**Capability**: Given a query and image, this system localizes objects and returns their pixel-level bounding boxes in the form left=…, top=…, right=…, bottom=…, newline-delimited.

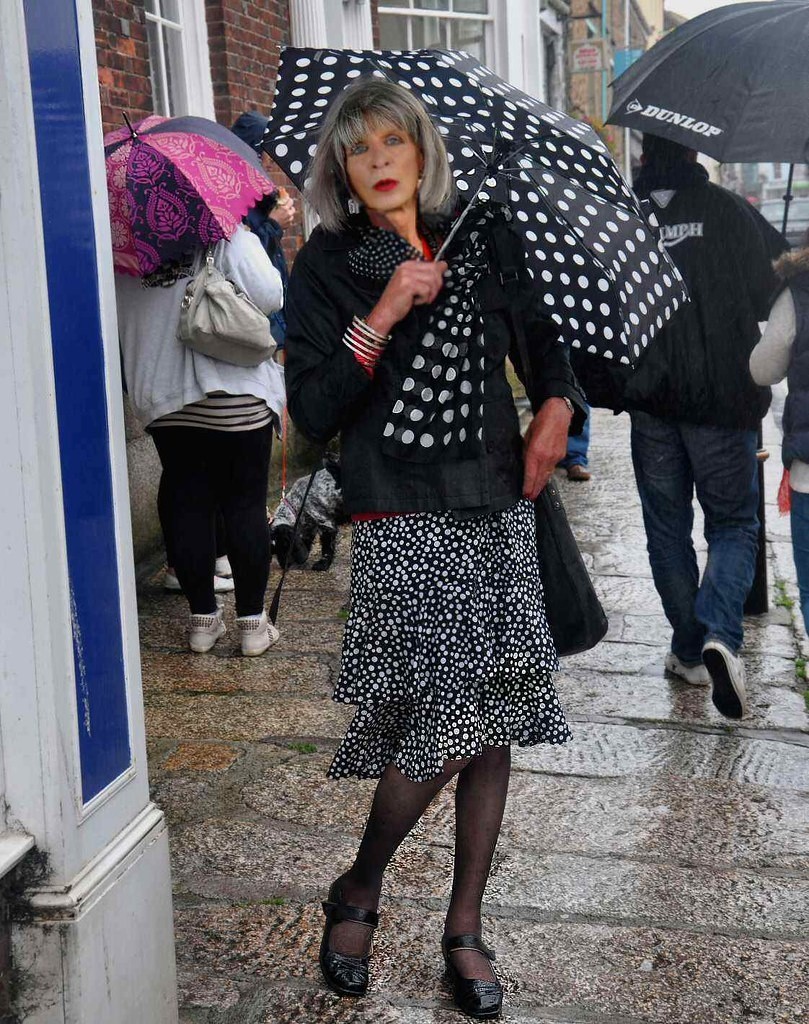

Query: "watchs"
left=564, top=397, right=574, bottom=416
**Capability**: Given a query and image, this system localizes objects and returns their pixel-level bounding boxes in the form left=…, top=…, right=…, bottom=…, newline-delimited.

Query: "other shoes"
left=163, top=554, right=235, bottom=592
left=567, top=464, right=591, bottom=481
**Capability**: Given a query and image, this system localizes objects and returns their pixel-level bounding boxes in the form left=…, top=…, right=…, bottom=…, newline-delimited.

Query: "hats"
left=230, top=111, right=270, bottom=157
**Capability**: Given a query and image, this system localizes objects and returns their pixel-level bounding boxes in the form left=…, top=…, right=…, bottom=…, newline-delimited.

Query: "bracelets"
left=343, top=315, right=391, bottom=367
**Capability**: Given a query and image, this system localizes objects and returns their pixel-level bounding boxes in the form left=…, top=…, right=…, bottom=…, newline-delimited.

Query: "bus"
left=761, top=180, right=809, bottom=201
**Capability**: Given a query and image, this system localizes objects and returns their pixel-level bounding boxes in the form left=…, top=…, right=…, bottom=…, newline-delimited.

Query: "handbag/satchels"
left=176, top=241, right=276, bottom=368
left=532, top=474, right=608, bottom=657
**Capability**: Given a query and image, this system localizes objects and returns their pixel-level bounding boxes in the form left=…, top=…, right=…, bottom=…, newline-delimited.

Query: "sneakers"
left=664, top=651, right=711, bottom=686
left=702, top=642, right=746, bottom=720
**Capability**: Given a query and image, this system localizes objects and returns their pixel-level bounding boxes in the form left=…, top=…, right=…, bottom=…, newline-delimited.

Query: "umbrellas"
left=256, top=45, right=693, bottom=372
left=601, top=0, right=808, bottom=236
left=103, top=115, right=274, bottom=285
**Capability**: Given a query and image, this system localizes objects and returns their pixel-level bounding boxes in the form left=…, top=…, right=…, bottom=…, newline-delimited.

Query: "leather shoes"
left=318, top=874, right=379, bottom=998
left=441, top=933, right=504, bottom=1019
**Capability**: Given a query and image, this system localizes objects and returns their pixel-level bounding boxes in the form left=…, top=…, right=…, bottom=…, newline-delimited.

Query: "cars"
left=758, top=199, right=809, bottom=248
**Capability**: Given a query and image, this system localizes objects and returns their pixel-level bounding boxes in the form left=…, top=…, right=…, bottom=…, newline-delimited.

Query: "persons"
left=117, top=111, right=296, bottom=656
left=750, top=228, right=809, bottom=635
left=556, top=406, right=590, bottom=480
left=628, top=132, right=792, bottom=719
left=284, top=84, right=590, bottom=1019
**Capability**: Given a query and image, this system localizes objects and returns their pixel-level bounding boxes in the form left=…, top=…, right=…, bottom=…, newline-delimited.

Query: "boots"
left=234, top=609, right=280, bottom=657
left=189, top=609, right=226, bottom=652
